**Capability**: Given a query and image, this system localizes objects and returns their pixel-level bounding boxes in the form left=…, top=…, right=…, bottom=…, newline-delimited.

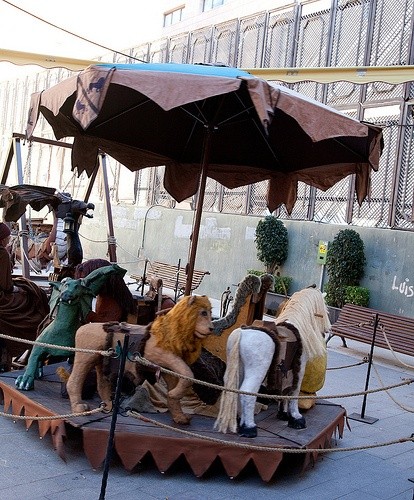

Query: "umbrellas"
left=26, top=63, right=385, bottom=296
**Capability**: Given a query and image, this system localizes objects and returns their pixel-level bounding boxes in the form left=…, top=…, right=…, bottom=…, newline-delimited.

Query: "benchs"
left=326, top=303, right=414, bottom=357
left=130, top=259, right=210, bottom=299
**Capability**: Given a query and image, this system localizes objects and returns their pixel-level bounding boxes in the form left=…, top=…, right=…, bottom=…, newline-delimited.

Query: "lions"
left=57, top=295, right=215, bottom=425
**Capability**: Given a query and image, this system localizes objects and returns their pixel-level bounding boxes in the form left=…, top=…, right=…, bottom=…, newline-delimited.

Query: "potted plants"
left=233, top=215, right=370, bottom=325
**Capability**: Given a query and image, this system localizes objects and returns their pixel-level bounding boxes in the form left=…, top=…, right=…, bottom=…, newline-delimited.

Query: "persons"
left=0, top=222, right=50, bottom=371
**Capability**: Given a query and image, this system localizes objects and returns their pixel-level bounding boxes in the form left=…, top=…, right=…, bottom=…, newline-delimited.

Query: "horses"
left=73, top=252, right=332, bottom=439
